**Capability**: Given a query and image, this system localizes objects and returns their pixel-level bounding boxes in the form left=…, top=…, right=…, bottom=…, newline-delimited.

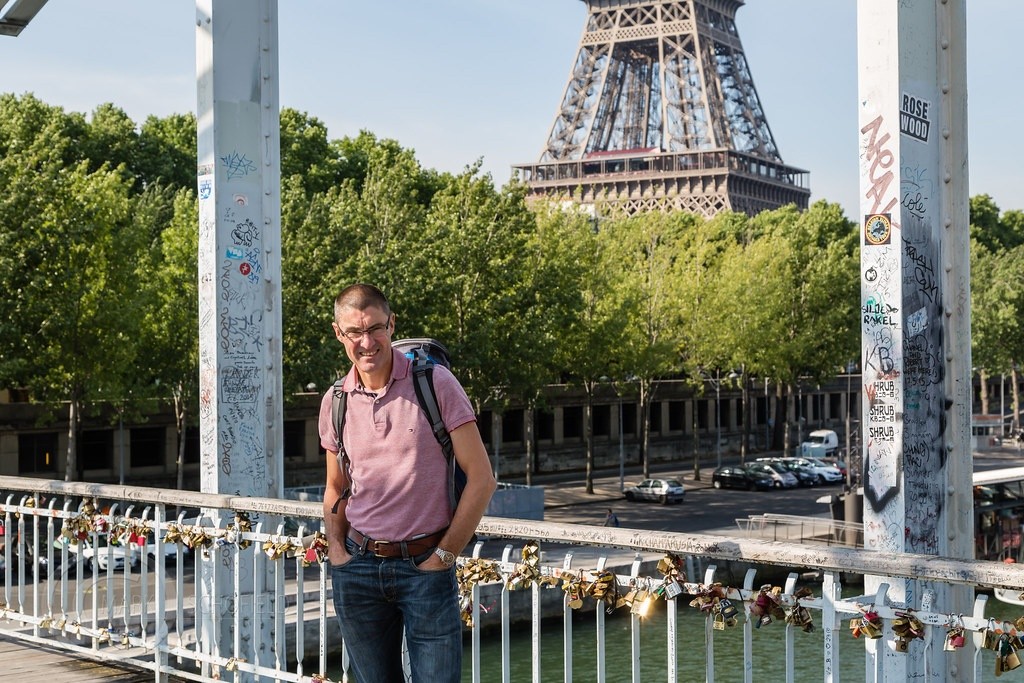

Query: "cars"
left=712, top=456, right=857, bottom=491
left=0, top=520, right=191, bottom=582
left=622, top=478, right=686, bottom=505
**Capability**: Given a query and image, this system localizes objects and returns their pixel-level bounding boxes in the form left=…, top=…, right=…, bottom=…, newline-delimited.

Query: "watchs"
left=434, top=547, right=456, bottom=568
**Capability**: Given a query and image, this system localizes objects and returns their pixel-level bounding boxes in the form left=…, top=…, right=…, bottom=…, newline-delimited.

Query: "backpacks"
left=331, top=337, right=468, bottom=516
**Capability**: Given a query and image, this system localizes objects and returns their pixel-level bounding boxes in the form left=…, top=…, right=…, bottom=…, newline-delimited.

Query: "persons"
left=605, top=508, right=618, bottom=528
left=317, top=284, right=499, bottom=683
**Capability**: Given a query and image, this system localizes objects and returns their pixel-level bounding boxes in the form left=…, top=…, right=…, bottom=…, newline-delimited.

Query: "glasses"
left=335, top=313, right=392, bottom=342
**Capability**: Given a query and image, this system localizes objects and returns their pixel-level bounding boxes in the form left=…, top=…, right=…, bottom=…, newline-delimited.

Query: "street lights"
left=698, top=370, right=738, bottom=469
left=599, top=376, right=645, bottom=493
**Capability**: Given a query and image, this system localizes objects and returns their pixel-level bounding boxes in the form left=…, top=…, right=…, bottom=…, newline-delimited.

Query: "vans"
left=806, top=429, right=839, bottom=455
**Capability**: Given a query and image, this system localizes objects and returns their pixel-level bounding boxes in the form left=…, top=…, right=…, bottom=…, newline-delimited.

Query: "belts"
left=347, top=525, right=449, bottom=558
